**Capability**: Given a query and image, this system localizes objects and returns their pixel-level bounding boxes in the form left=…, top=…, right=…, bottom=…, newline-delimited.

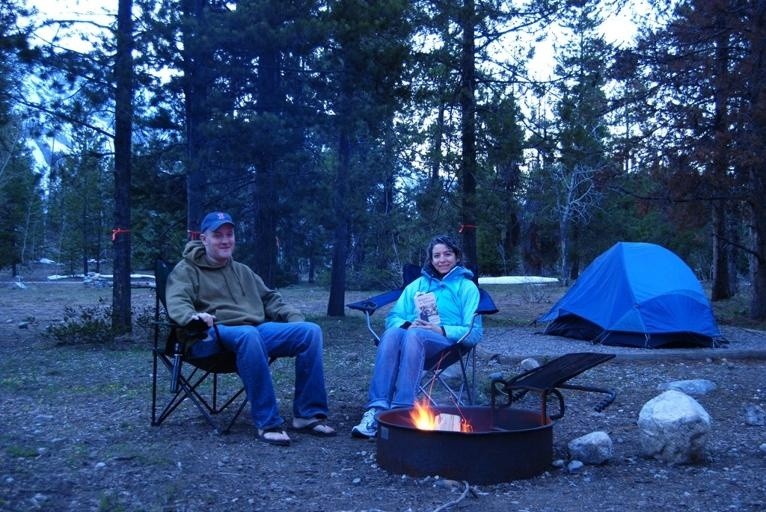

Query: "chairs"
left=345, top=262, right=498, bottom=407
left=149, top=252, right=278, bottom=437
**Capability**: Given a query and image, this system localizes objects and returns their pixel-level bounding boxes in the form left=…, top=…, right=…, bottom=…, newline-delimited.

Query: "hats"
left=200, top=211, right=235, bottom=233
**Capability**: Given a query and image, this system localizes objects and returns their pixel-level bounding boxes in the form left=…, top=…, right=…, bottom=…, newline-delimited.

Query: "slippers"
left=257, top=426, right=292, bottom=446
left=287, top=417, right=338, bottom=438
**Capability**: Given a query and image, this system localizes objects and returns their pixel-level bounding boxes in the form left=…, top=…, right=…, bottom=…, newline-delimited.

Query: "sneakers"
left=350, top=407, right=379, bottom=440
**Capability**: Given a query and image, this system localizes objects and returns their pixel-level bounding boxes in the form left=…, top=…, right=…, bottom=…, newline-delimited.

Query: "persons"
left=351, top=235, right=484, bottom=439
left=165, top=212, right=338, bottom=448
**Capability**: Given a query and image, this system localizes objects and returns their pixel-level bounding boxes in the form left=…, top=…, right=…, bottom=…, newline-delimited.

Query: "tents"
left=528, top=242, right=730, bottom=351
